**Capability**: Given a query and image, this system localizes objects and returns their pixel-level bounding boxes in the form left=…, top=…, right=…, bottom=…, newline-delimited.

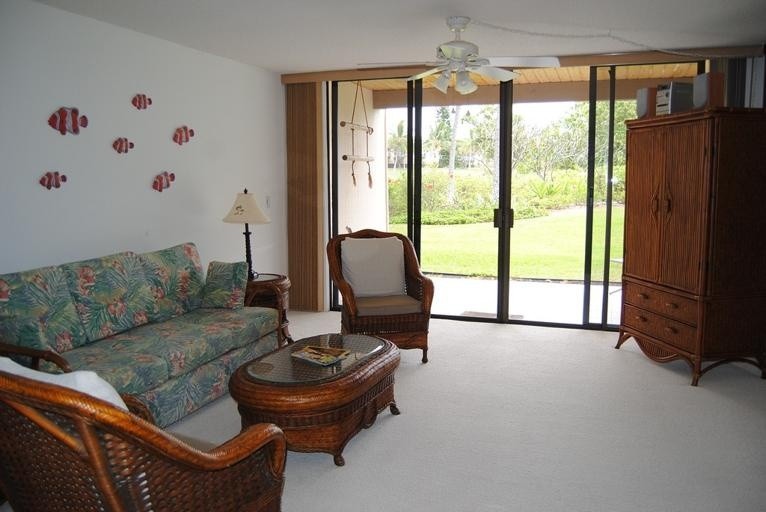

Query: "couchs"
left=0, top=282, right=290, bottom=429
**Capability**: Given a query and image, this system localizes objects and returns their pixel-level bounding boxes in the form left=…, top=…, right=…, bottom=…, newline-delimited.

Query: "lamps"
left=407, top=17, right=520, bottom=96
left=223, top=188, right=271, bottom=281
left=440, top=16, right=480, bottom=63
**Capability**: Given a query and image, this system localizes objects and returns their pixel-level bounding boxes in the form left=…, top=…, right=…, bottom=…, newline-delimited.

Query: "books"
left=292, top=346, right=351, bottom=367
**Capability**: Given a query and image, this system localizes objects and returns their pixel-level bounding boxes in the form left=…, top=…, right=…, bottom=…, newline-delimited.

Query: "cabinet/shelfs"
left=614, top=107, right=766, bottom=386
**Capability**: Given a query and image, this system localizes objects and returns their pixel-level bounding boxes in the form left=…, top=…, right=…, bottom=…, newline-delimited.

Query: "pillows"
left=137, top=242, right=206, bottom=324
left=340, top=236, right=406, bottom=299
left=1, top=356, right=154, bottom=508
left=60, top=251, right=162, bottom=345
left=0, top=264, right=87, bottom=368
left=201, top=261, right=249, bottom=310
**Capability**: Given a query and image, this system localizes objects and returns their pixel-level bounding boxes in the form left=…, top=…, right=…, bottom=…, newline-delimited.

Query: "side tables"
left=247, top=273, right=295, bottom=344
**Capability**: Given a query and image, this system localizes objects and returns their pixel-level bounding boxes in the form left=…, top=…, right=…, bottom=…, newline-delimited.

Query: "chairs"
left=326, top=229, right=434, bottom=363
left=0, top=371, right=287, bottom=512
